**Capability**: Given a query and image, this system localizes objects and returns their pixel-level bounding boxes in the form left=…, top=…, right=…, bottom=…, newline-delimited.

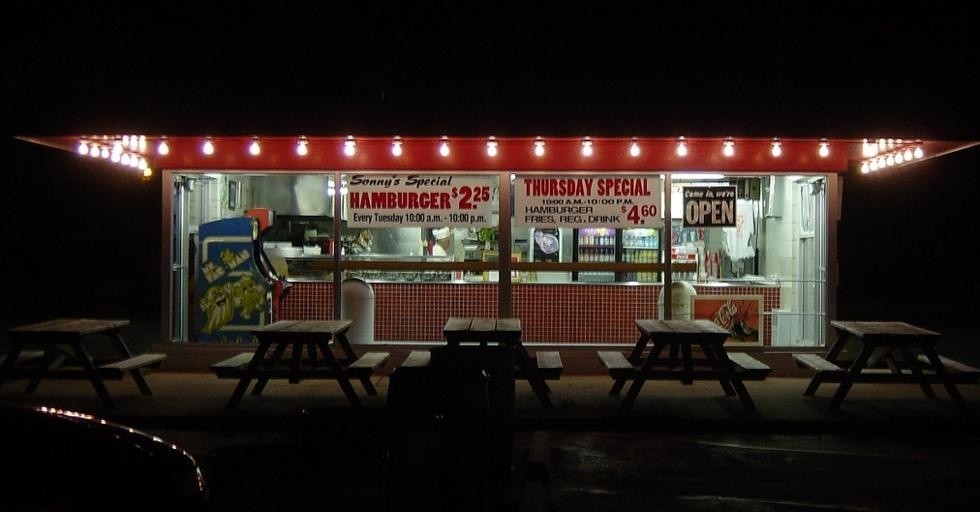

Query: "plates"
left=263, top=242, right=321, bottom=257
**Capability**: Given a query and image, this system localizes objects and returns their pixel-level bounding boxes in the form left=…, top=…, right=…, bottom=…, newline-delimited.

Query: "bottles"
left=579, top=248, right=659, bottom=264
left=579, top=228, right=657, bottom=249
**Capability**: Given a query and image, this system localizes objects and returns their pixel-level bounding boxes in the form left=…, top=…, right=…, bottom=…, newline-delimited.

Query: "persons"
left=428, top=228, right=451, bottom=256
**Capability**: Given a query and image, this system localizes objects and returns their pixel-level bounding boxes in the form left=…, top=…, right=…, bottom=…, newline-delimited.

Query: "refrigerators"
left=571, top=225, right=662, bottom=284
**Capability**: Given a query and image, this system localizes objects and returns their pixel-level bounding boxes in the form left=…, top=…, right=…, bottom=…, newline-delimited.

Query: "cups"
left=705, top=249, right=718, bottom=278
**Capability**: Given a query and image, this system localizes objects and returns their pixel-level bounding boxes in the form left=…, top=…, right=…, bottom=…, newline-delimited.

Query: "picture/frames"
left=690, top=294, right=764, bottom=347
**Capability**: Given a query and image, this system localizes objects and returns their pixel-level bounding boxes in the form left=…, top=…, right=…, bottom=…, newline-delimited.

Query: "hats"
left=433, top=227, right=450, bottom=240
